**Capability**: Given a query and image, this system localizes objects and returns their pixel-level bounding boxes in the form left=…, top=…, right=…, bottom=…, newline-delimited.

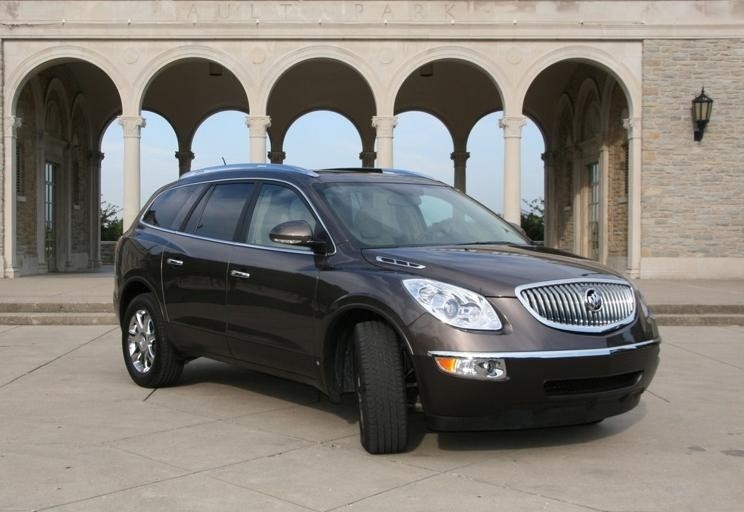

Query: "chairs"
left=353, top=208, right=407, bottom=247
left=253, top=205, right=320, bottom=250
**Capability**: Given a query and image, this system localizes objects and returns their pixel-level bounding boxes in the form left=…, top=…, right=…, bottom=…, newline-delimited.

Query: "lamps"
left=419, top=63, right=434, bottom=76
left=692, top=85, right=714, bottom=141
left=209, top=62, right=222, bottom=76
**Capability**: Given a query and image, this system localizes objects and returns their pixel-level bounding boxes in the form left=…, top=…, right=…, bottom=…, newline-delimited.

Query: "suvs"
left=113, top=158, right=660, bottom=456
